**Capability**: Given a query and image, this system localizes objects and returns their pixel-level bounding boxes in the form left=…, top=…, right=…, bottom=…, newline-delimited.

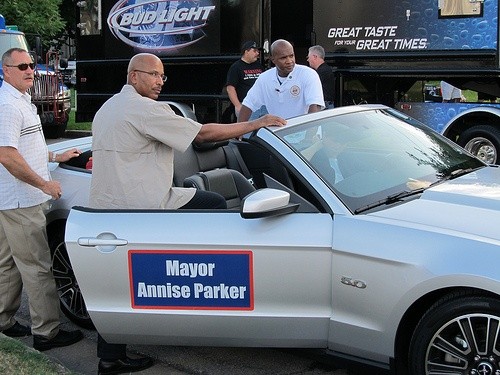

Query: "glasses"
left=134, top=69, right=167, bottom=82
left=6, top=63, right=36, bottom=71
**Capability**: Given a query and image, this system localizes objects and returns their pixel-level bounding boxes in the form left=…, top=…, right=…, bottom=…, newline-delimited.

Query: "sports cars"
left=45, top=103, right=500, bottom=375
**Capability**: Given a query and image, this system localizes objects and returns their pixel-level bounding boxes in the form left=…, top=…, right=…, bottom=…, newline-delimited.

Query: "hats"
left=240, top=41, right=266, bottom=52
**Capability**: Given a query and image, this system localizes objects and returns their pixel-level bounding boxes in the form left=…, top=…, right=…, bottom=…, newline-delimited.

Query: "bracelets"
left=52, top=152, right=57, bottom=163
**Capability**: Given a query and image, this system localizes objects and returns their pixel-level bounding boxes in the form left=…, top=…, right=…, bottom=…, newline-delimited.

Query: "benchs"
left=169, top=142, right=259, bottom=198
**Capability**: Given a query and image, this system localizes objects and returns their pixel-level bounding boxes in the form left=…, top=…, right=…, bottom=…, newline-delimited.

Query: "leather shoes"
left=33, top=329, right=83, bottom=352
left=2, top=321, right=32, bottom=337
left=97, top=354, right=154, bottom=375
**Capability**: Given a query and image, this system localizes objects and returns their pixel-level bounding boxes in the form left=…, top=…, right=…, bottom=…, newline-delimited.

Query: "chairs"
left=183, top=168, right=258, bottom=210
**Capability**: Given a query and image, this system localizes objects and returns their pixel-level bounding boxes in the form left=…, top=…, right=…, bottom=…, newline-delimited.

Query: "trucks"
left=71, top=0, right=500, bottom=165
left=0, top=13, right=72, bottom=139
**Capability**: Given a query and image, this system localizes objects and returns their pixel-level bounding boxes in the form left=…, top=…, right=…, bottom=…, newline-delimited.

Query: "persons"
left=441, top=81, right=466, bottom=103
left=307, top=45, right=335, bottom=110
left=0, top=48, right=83, bottom=351
left=231, top=39, right=326, bottom=143
left=89, top=53, right=287, bottom=375
left=225, top=40, right=265, bottom=123
